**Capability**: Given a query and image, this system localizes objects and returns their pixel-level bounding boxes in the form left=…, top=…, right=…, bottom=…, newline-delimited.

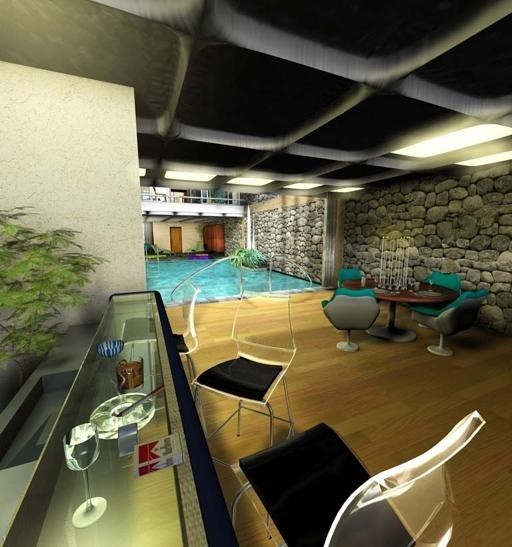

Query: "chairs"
left=122, top=278, right=202, bottom=408
left=150, top=244, right=177, bottom=255
left=185, top=239, right=201, bottom=255
left=320, top=285, right=380, bottom=354
left=152, top=186, right=166, bottom=201
left=398, top=270, right=462, bottom=328
left=410, top=286, right=489, bottom=358
left=335, top=266, right=367, bottom=289
left=188, top=286, right=301, bottom=470
left=230, top=407, right=488, bottom=547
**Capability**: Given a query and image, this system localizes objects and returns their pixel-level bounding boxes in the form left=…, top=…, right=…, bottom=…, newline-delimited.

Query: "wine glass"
left=62, top=422, right=109, bottom=528
left=408, top=278, right=415, bottom=290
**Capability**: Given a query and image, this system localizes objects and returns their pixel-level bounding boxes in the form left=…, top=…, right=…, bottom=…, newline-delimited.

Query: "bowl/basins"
left=96, top=339, right=124, bottom=357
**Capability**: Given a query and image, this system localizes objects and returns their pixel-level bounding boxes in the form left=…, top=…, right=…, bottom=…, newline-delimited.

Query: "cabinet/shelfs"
left=203, top=222, right=227, bottom=257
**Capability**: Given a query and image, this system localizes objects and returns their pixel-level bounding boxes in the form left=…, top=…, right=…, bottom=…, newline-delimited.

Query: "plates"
left=89, top=392, right=156, bottom=439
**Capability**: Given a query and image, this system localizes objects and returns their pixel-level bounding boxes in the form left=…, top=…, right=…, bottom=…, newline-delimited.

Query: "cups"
left=115, top=354, right=145, bottom=394
left=414, top=281, right=420, bottom=291
left=360, top=276, right=366, bottom=287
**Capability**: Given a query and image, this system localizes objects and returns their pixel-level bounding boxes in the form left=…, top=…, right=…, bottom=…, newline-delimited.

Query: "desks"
left=341, top=277, right=460, bottom=346
